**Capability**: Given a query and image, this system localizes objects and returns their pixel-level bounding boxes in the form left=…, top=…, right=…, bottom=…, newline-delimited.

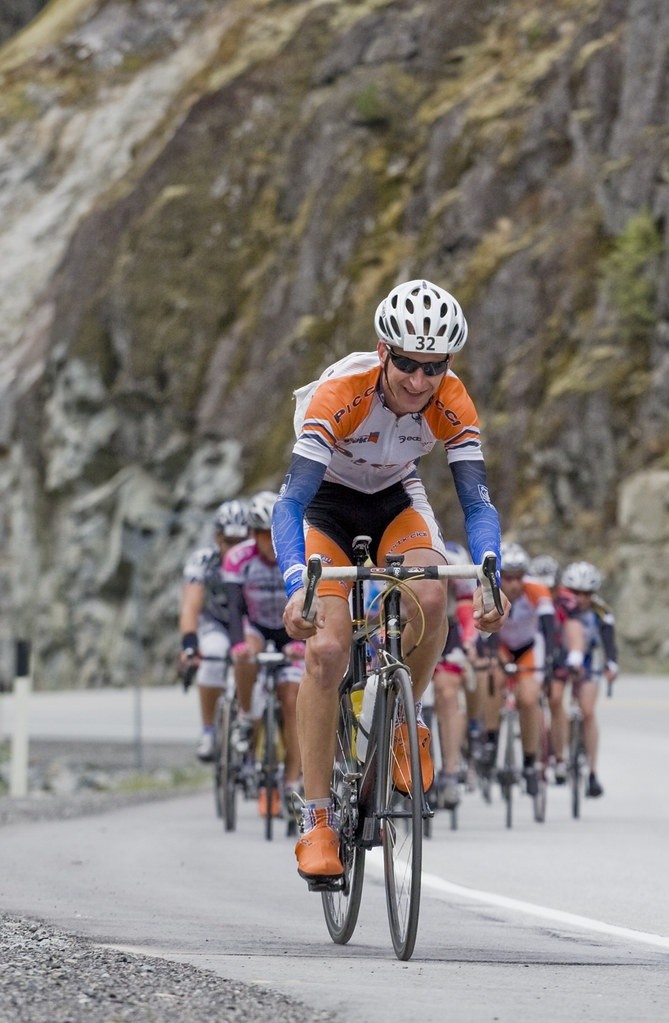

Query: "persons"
left=179, top=493, right=618, bottom=834
left=269, top=276, right=512, bottom=885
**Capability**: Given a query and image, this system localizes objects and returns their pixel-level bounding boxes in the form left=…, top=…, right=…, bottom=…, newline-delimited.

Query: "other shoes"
left=258, top=784, right=280, bottom=816
left=589, top=777, right=602, bottom=795
left=294, top=811, right=345, bottom=876
left=230, top=723, right=251, bottom=751
left=396, top=715, right=435, bottom=794
left=200, top=733, right=218, bottom=757
left=437, top=778, right=459, bottom=806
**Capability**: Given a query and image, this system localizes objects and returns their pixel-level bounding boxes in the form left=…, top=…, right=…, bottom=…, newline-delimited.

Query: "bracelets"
left=182, top=633, right=197, bottom=650
left=564, top=650, right=583, bottom=669
left=607, top=660, right=618, bottom=673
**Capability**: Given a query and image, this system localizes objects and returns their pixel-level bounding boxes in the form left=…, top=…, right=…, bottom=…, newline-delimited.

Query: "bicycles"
left=181, top=652, right=616, bottom=840
left=301, top=533, right=503, bottom=960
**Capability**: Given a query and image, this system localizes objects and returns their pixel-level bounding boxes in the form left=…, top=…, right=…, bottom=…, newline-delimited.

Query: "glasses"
left=387, top=343, right=450, bottom=377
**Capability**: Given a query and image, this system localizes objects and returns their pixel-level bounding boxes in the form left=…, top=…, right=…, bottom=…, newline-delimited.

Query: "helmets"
left=445, top=543, right=602, bottom=591
left=214, top=499, right=248, bottom=534
left=247, top=490, right=278, bottom=529
left=375, top=278, right=469, bottom=355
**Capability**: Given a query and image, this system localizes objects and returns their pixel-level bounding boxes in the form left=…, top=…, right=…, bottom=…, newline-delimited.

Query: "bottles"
left=350, top=674, right=379, bottom=762
